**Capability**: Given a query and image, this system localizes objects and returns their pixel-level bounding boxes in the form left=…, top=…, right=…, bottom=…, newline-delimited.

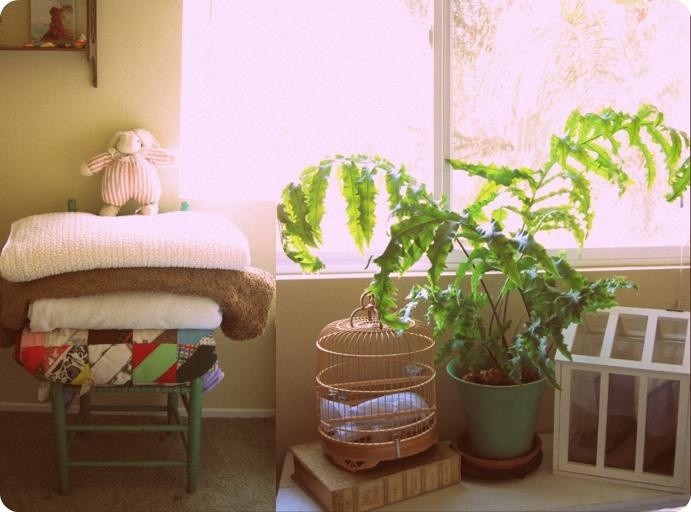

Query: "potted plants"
left=278, top=102, right=691, bottom=460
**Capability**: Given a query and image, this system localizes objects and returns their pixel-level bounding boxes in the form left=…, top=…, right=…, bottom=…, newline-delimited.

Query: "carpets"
left=0, top=411, right=277, bottom=512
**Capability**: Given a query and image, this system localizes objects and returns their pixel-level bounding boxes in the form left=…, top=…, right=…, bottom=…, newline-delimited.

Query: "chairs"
left=49, top=198, right=203, bottom=495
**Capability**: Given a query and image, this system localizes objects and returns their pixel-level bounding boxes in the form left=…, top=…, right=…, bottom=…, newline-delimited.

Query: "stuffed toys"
left=77, top=125, right=177, bottom=216
